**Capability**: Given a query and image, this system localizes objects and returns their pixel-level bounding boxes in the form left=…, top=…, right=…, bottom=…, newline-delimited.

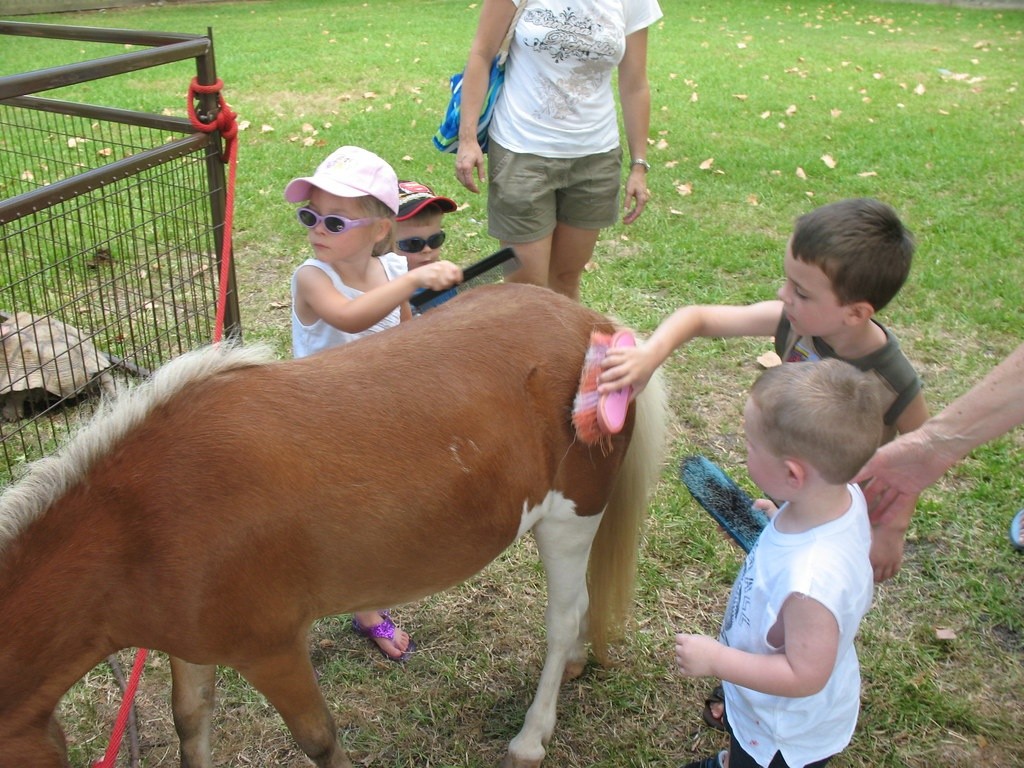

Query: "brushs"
left=409, top=246, right=523, bottom=315
left=679, top=454, right=771, bottom=554
left=571, top=329, right=638, bottom=453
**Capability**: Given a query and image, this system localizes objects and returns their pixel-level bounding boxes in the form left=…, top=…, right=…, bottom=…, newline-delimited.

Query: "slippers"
left=351, top=609, right=415, bottom=660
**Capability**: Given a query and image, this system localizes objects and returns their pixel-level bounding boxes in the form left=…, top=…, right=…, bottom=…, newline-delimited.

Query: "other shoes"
left=704, top=682, right=728, bottom=731
left=679, top=749, right=729, bottom=768
left=1010, top=508, right=1024, bottom=549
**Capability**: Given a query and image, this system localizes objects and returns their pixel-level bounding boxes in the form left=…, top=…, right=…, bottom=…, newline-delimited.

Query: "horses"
left=1, top=284, right=672, bottom=768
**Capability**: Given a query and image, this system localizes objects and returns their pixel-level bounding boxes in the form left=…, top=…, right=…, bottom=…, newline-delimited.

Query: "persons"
left=596, top=197, right=930, bottom=585
left=291, top=145, right=463, bottom=660
left=680, top=357, right=874, bottom=768
left=850, top=341, right=1024, bottom=528
left=455, top=0, right=664, bottom=304
left=1009, top=509, right=1024, bottom=552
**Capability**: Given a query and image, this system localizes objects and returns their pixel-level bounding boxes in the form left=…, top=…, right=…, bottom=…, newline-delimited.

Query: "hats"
left=394, top=180, right=457, bottom=221
left=285, top=146, right=399, bottom=215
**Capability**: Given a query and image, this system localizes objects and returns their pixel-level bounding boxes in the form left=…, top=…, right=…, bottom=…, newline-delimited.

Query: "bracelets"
left=630, top=159, right=651, bottom=173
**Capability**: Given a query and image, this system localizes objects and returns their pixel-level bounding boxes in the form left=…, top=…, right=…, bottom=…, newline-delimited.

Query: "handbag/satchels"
left=432, top=0, right=531, bottom=154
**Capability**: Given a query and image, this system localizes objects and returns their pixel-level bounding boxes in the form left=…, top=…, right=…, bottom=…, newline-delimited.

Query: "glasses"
left=296, top=204, right=380, bottom=234
left=394, top=230, right=446, bottom=253
